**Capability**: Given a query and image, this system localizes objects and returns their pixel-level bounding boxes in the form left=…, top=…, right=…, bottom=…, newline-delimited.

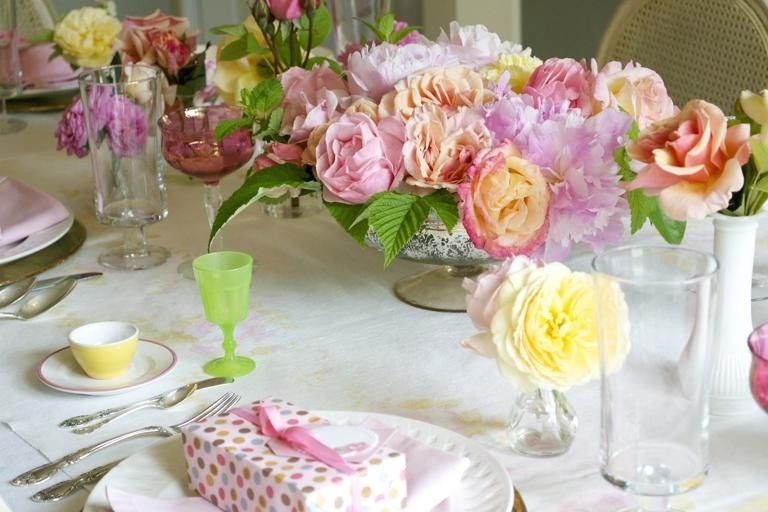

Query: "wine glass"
left=75, top=64, right=175, bottom=272
left=191, top=250, right=257, bottom=377
left=158, top=102, right=254, bottom=279
left=0, top=0, right=26, bottom=137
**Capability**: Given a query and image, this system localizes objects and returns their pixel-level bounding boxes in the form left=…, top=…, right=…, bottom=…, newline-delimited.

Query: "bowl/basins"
left=66, top=320, right=136, bottom=380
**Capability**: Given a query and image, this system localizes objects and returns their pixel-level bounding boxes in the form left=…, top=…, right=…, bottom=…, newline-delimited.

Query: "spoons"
left=0, top=277, right=81, bottom=324
left=75, top=381, right=201, bottom=435
left=0, top=274, right=40, bottom=307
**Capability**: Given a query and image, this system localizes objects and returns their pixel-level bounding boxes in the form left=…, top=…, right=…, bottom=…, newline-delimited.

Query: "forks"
left=12, top=390, right=240, bottom=489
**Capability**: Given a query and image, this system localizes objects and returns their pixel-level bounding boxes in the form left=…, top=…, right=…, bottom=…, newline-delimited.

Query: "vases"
left=501, top=377, right=582, bottom=459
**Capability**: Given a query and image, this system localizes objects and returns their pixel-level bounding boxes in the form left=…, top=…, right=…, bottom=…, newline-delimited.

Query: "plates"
left=7, top=79, right=96, bottom=105
left=1, top=200, right=72, bottom=266
left=37, top=339, right=179, bottom=396
left=81, top=404, right=515, bottom=512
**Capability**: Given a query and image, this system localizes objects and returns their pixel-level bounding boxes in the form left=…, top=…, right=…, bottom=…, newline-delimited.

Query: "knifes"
left=32, top=271, right=107, bottom=291
left=59, top=376, right=235, bottom=430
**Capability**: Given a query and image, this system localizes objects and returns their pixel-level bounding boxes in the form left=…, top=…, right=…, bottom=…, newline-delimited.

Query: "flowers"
left=462, top=252, right=633, bottom=393
left=52, top=0, right=766, bottom=266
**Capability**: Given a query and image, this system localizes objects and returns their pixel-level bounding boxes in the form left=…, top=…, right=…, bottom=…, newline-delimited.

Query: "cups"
left=746, top=319, right=768, bottom=413
left=592, top=242, right=722, bottom=499
